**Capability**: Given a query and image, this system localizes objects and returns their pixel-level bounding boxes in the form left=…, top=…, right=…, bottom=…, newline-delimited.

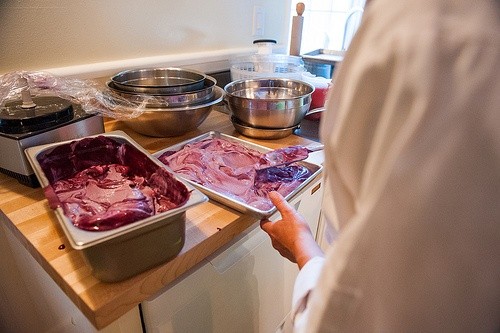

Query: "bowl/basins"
left=95, top=67, right=225, bottom=137
left=24, top=129, right=209, bottom=283
left=224, top=77, right=316, bottom=129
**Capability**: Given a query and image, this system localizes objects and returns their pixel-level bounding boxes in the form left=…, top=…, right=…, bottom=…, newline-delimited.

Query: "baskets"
left=230, top=61, right=306, bottom=81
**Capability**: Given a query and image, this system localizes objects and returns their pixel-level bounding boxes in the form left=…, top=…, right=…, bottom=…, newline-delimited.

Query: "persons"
left=260, top=0, right=500, bottom=333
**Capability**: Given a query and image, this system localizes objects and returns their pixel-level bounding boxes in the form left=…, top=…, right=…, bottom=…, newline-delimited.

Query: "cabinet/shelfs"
left=0, top=162, right=324, bottom=333
